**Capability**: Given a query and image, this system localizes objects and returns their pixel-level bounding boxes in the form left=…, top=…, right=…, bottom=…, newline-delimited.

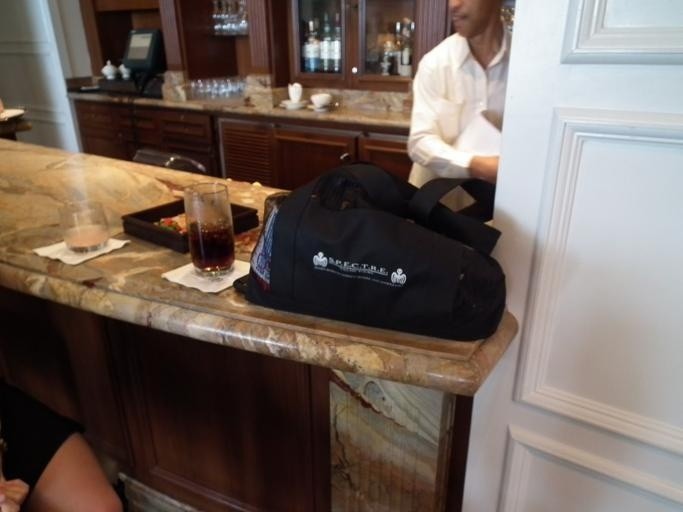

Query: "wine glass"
left=210, top=0, right=248, bottom=35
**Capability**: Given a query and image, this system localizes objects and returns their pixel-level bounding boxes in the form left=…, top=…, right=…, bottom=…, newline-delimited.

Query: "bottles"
left=319, top=12, right=331, bottom=72
left=303, top=20, right=319, bottom=70
left=330, top=14, right=341, bottom=72
left=100, top=60, right=117, bottom=80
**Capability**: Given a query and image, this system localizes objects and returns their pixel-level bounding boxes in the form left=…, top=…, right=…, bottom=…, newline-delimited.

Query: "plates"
left=281, top=99, right=307, bottom=109
left=308, top=105, right=332, bottom=112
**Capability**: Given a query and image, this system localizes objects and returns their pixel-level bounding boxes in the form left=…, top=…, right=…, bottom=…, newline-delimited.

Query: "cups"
left=311, top=94, right=329, bottom=107
left=184, top=183, right=240, bottom=277
left=188, top=76, right=244, bottom=98
left=118, top=63, right=131, bottom=79
left=59, top=202, right=109, bottom=252
left=288, top=83, right=302, bottom=102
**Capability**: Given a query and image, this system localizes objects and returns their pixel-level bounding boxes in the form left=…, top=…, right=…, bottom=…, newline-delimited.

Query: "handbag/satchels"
left=246, top=160, right=505, bottom=340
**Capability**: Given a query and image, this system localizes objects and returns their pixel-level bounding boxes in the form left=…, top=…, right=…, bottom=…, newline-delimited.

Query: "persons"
left=1, top=375, right=125, bottom=512
left=403, top=1, right=513, bottom=222
